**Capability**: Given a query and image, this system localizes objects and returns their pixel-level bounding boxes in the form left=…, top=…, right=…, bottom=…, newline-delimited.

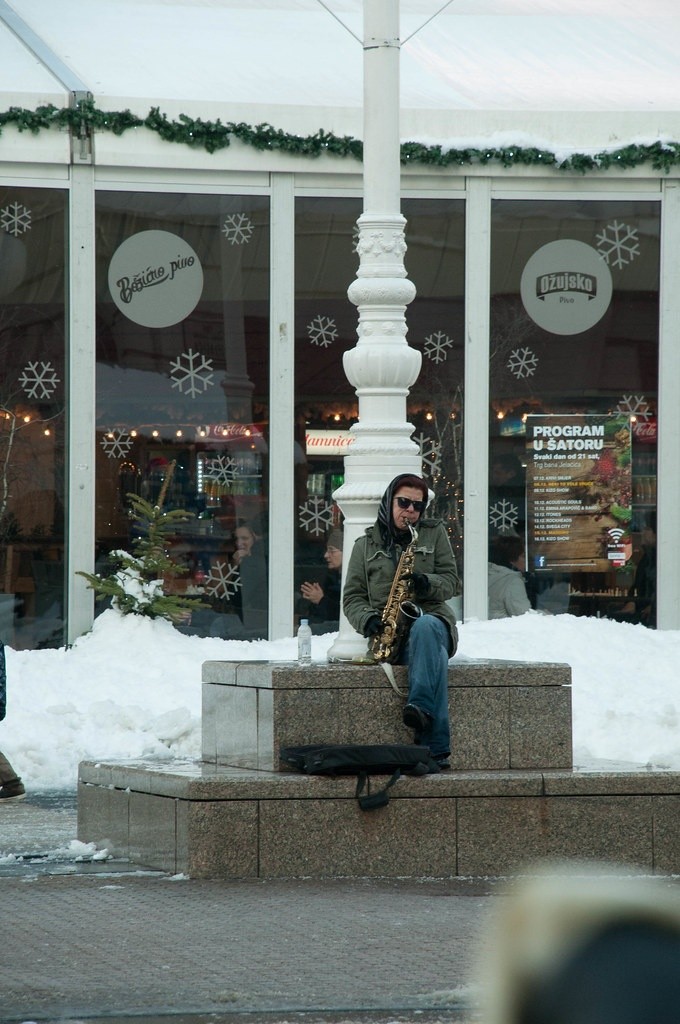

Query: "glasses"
left=393, top=496, right=425, bottom=512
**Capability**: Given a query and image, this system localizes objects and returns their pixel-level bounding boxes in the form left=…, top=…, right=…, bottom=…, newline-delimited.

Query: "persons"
left=614, top=510, right=657, bottom=630
left=487, top=534, right=536, bottom=621
left=230, top=522, right=268, bottom=570
left=0, top=639, right=25, bottom=800
left=343, top=472, right=458, bottom=769
left=463, top=859, right=680, bottom=1024
left=301, top=528, right=345, bottom=624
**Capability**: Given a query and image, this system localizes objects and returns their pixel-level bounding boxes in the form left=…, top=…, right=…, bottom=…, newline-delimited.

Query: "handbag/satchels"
left=279, top=743, right=432, bottom=809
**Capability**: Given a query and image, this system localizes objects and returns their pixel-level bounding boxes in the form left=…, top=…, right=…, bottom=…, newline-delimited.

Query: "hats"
left=326, top=529, right=343, bottom=550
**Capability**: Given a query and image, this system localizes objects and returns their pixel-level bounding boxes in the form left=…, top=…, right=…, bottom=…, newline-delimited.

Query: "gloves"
left=399, top=573, right=429, bottom=597
left=364, top=618, right=385, bottom=638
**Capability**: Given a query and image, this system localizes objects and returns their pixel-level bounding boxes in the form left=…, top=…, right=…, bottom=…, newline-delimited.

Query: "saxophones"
left=370, top=517, right=425, bottom=660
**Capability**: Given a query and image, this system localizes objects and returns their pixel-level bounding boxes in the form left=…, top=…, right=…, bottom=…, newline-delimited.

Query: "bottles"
left=297, top=619, right=312, bottom=666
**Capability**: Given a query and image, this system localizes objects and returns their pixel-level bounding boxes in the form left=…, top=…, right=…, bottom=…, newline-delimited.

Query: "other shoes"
left=0, top=777, right=26, bottom=798
left=403, top=703, right=430, bottom=734
left=433, top=755, right=451, bottom=769
